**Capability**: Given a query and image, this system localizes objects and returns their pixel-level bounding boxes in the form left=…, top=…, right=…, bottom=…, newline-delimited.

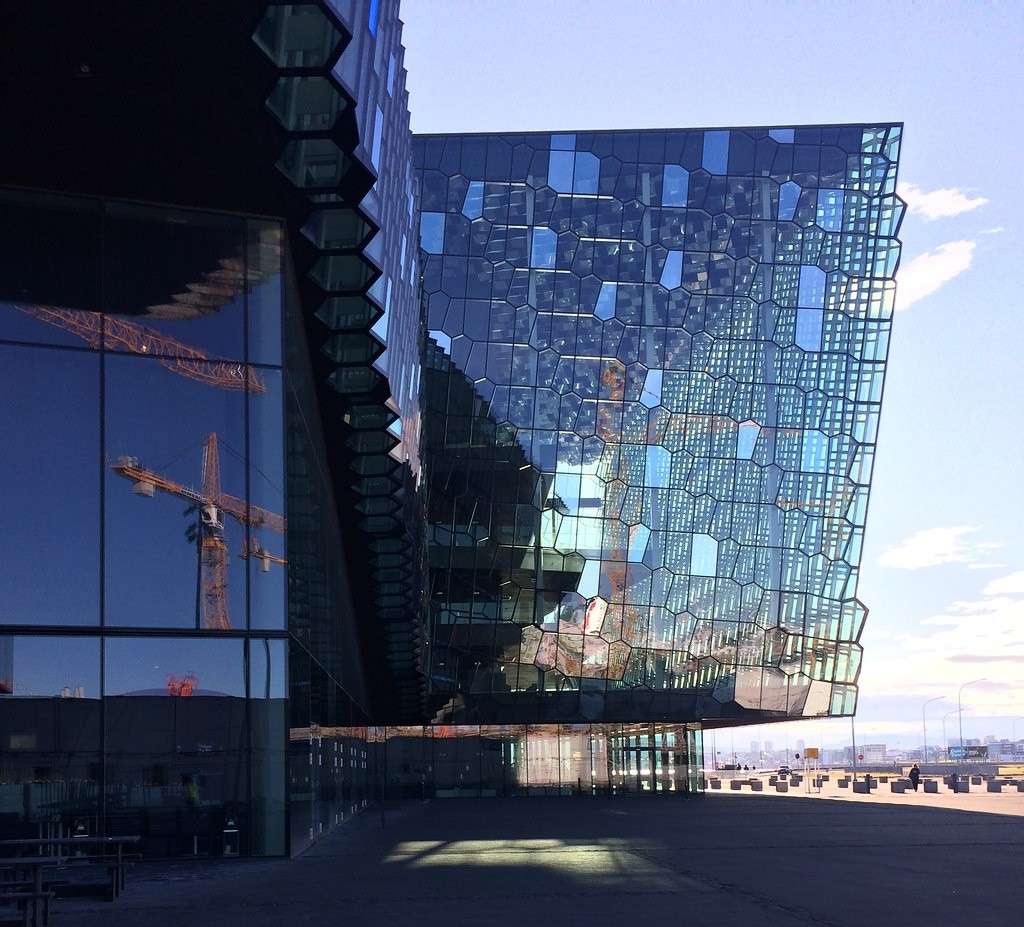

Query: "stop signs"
left=858, top=755, right=864, bottom=760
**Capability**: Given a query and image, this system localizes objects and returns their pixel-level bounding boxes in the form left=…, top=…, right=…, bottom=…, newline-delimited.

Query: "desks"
left=0, top=856, right=67, bottom=922
left=0, top=835, right=141, bottom=898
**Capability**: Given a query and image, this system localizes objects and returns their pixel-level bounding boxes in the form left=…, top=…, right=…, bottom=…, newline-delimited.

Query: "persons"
left=744, top=765, right=749, bottom=770
left=909, top=764, right=920, bottom=792
left=737, top=763, right=741, bottom=770
left=182, top=773, right=201, bottom=807
left=752, top=765, right=756, bottom=770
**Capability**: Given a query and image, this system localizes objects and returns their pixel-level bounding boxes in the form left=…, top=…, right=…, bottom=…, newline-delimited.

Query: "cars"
left=778, top=765, right=793, bottom=775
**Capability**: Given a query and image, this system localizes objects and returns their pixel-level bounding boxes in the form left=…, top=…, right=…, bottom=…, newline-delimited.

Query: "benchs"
left=1, top=861, right=135, bottom=903
left=0, top=876, right=72, bottom=916
left=66, top=853, right=143, bottom=891
left=0, top=891, right=55, bottom=926
left=435, top=787, right=497, bottom=797
left=518, top=787, right=572, bottom=796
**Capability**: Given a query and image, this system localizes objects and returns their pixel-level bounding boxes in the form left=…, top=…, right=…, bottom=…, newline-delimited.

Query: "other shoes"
left=913, top=787, right=917, bottom=792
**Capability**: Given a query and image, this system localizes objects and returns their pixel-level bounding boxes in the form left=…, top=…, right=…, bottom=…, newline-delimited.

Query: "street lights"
left=922, top=696, right=945, bottom=763
left=943, top=709, right=965, bottom=762
left=959, top=679, right=988, bottom=763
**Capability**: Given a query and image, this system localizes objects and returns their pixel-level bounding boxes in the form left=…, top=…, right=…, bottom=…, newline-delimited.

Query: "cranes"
left=108, top=430, right=282, bottom=629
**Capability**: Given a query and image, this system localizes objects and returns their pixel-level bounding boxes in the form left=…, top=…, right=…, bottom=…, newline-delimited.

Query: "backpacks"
left=909, top=772, right=912, bottom=779
